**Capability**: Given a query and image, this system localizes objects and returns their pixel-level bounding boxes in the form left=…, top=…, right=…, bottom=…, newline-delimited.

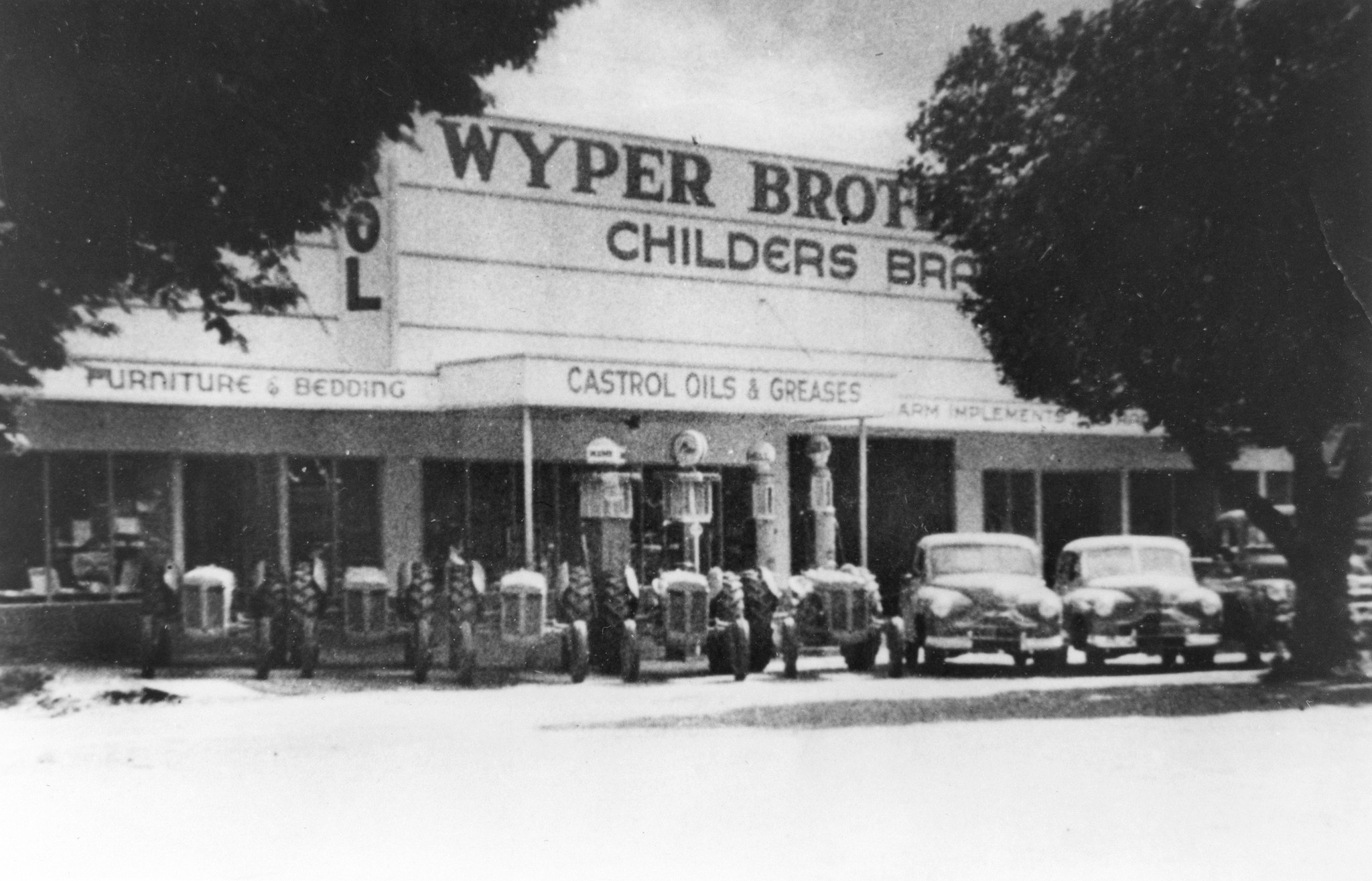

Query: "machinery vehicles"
left=136, top=559, right=903, bottom=683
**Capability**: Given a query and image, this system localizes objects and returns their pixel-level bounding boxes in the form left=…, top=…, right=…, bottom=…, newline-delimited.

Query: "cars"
left=1200, top=551, right=1372, bottom=648
left=901, top=528, right=1072, bottom=675
left=1056, top=531, right=1226, bottom=676
